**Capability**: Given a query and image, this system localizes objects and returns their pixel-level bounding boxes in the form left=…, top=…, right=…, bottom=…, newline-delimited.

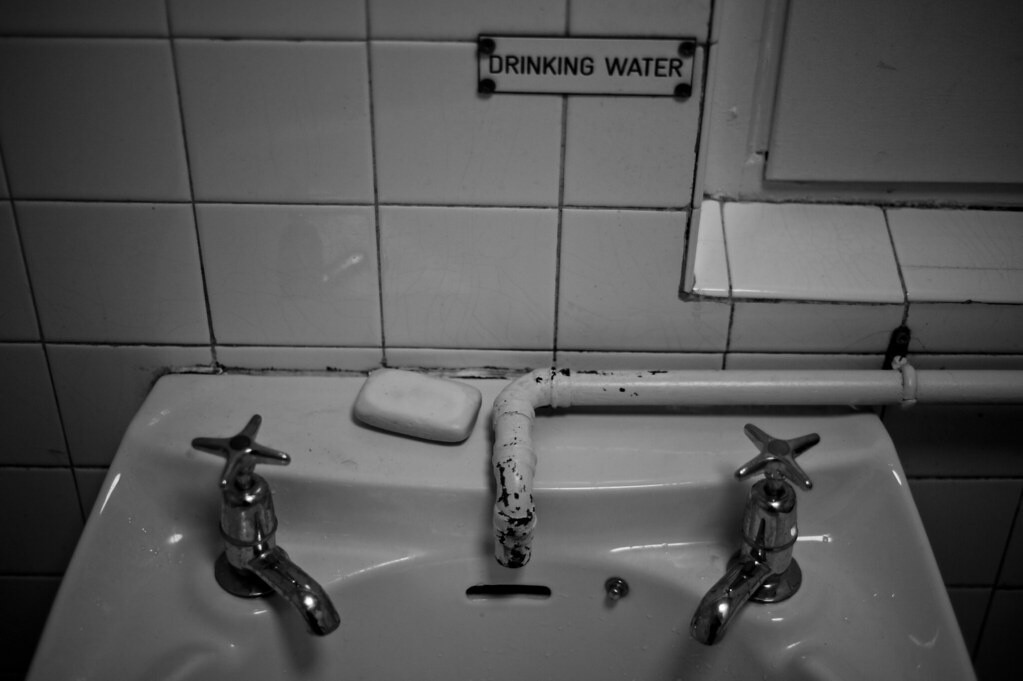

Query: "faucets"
left=689, top=422, right=821, bottom=647
left=191, top=413, right=342, bottom=638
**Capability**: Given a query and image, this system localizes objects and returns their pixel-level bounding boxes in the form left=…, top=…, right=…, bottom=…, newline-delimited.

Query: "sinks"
left=170, top=548, right=837, bottom=681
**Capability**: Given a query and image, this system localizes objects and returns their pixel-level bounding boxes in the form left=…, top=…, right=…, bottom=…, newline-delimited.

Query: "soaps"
left=352, top=367, right=483, bottom=444
